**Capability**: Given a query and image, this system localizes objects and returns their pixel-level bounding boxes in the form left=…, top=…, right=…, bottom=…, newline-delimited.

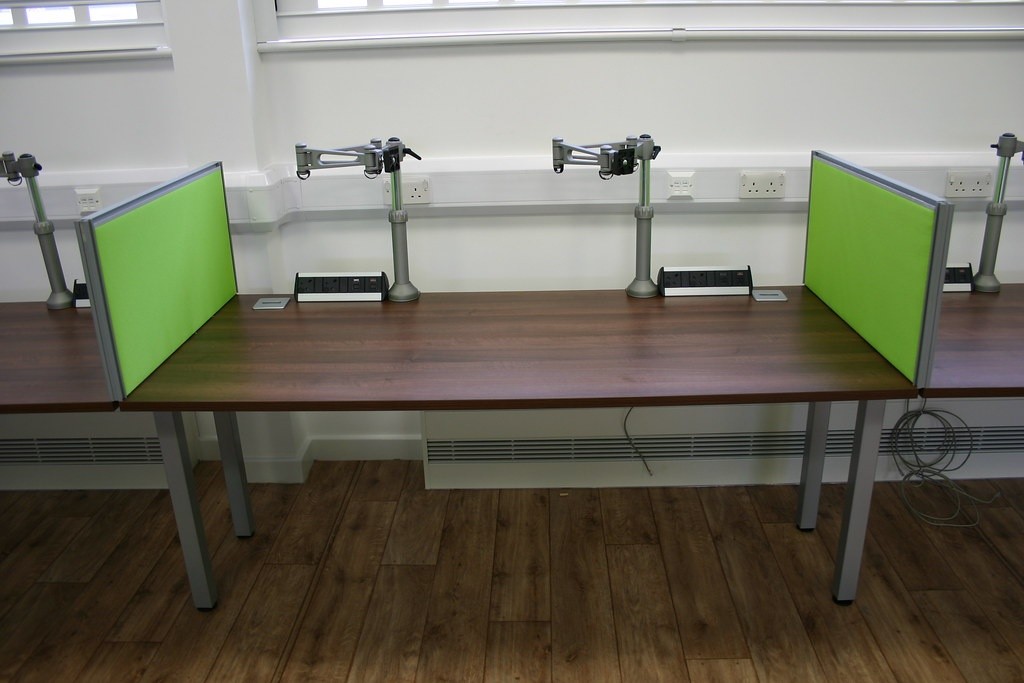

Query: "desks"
left=1, top=284, right=1024, bottom=602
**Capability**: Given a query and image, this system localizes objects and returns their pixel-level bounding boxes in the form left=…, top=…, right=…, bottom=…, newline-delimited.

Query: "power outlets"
left=945, top=171, right=993, bottom=196
left=739, top=170, right=787, bottom=197
left=384, top=176, right=431, bottom=203
left=74, top=189, right=102, bottom=216
left=666, top=170, right=695, bottom=199
